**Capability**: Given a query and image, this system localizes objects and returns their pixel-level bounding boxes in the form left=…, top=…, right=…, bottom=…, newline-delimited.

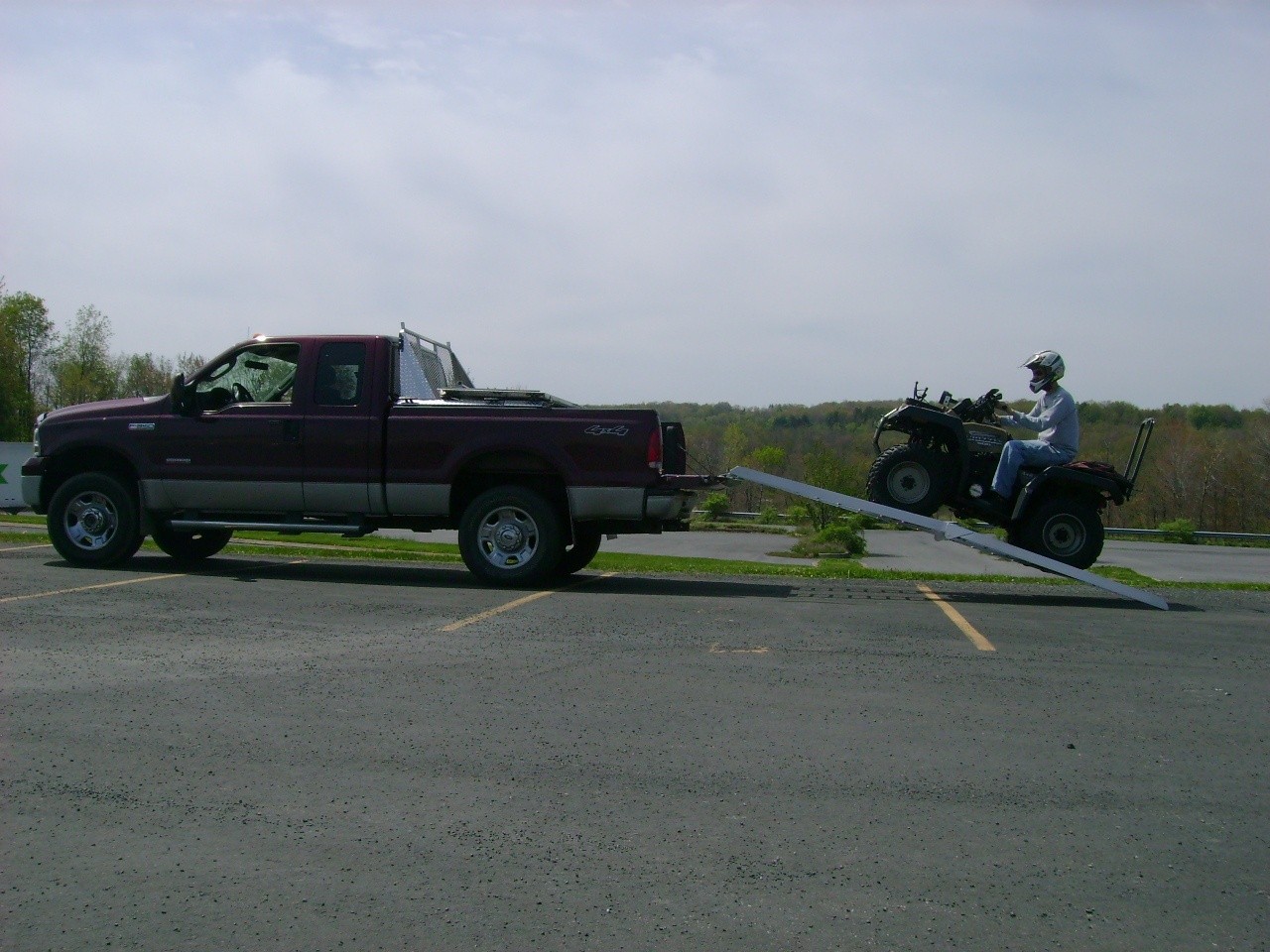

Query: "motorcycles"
left=865, top=381, right=1157, bottom=570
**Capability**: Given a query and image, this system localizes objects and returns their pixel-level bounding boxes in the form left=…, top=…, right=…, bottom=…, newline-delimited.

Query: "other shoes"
left=974, top=488, right=1007, bottom=510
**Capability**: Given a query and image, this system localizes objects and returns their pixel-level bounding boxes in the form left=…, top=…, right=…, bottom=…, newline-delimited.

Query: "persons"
left=976, top=349, right=1079, bottom=507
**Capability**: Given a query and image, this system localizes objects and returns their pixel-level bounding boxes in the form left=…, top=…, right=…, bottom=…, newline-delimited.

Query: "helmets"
left=1024, top=350, right=1065, bottom=394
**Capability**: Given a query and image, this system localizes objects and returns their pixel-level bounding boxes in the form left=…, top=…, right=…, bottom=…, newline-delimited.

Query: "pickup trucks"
left=24, top=320, right=745, bottom=587
